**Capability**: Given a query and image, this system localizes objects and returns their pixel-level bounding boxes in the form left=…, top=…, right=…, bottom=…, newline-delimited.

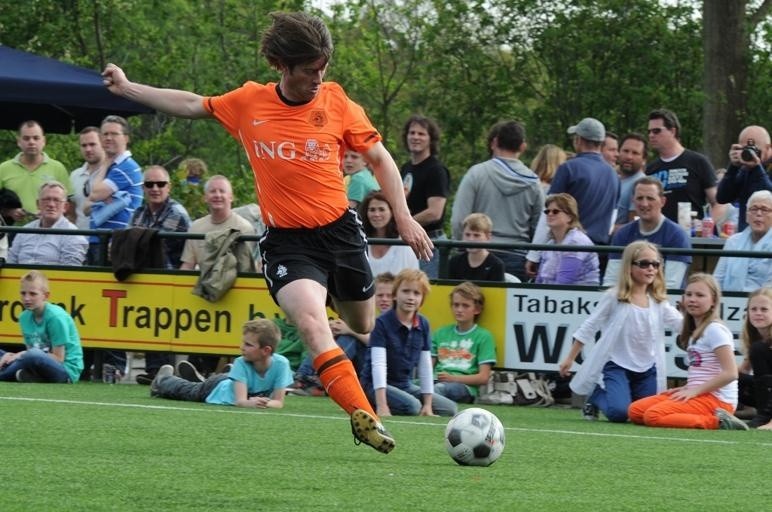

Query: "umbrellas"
left=0, top=44, right=156, bottom=134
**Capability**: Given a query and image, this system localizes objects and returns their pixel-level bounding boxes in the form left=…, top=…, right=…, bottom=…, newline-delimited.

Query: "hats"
left=568, top=117, right=607, bottom=143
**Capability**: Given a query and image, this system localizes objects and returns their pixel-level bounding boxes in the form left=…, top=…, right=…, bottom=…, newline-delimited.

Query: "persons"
left=99, top=11, right=435, bottom=454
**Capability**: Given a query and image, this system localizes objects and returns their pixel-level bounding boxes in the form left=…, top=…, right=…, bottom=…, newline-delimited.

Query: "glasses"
left=647, top=127, right=667, bottom=133
left=632, top=260, right=660, bottom=269
left=144, top=181, right=168, bottom=187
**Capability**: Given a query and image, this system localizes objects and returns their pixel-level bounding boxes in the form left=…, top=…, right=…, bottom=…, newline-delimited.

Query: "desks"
left=687, top=233, right=729, bottom=275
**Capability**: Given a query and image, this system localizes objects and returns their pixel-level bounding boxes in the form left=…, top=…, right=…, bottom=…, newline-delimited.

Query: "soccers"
left=444, top=408, right=506, bottom=467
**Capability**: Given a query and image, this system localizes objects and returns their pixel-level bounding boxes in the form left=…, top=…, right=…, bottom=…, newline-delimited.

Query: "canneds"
left=702, top=217, right=715, bottom=239
left=723, top=221, right=735, bottom=236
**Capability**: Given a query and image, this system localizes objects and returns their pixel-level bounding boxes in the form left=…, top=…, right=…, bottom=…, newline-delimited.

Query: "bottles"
left=90, top=196, right=132, bottom=227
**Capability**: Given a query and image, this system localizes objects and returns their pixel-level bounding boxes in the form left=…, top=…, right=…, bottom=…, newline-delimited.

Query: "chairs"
left=504, top=268, right=523, bottom=286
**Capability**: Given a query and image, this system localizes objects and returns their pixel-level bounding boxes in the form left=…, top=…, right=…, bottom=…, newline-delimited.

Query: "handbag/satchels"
left=472, top=365, right=556, bottom=408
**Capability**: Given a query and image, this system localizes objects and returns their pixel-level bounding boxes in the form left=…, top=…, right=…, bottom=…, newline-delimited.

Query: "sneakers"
left=714, top=407, right=749, bottom=431
left=150, top=364, right=172, bottom=395
left=179, top=360, right=206, bottom=385
left=13, top=367, right=42, bottom=381
left=582, top=383, right=599, bottom=422
left=351, top=408, right=396, bottom=453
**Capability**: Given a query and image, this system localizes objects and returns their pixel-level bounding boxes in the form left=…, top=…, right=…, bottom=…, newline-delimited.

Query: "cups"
left=677, top=201, right=738, bottom=240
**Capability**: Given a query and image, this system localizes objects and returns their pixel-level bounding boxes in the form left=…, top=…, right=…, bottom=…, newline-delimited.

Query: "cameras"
left=736, top=138, right=761, bottom=161
